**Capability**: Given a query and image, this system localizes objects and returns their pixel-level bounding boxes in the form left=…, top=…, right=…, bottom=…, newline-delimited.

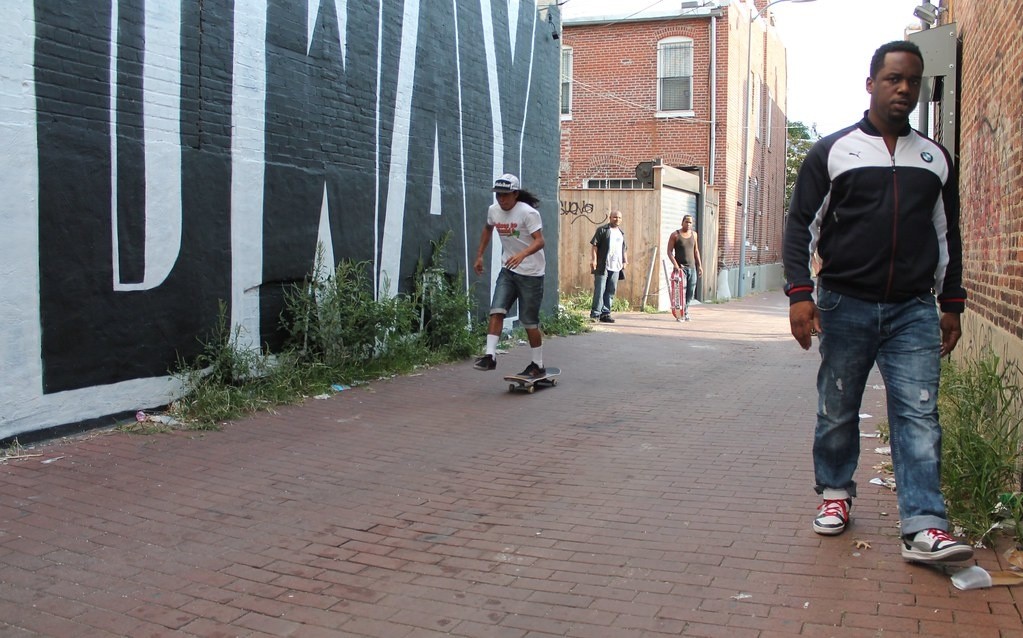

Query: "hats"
left=490, top=174, right=520, bottom=194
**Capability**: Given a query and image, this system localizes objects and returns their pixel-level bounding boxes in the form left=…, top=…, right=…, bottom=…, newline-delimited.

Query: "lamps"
left=913, top=2, right=948, bottom=26
left=548, top=13, right=560, bottom=39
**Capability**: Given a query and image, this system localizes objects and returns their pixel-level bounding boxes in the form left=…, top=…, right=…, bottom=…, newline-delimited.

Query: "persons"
left=474, top=173, right=547, bottom=378
left=780, top=42, right=975, bottom=563
left=667, top=215, right=703, bottom=323
left=590, top=211, right=629, bottom=323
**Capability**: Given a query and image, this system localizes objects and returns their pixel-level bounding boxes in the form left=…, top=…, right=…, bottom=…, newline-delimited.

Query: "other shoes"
left=677, top=315, right=691, bottom=322
left=590, top=315, right=599, bottom=323
left=600, top=314, right=614, bottom=323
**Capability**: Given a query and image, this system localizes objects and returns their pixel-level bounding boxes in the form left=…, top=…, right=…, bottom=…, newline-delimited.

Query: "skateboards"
left=504, top=367, right=562, bottom=392
left=670, top=269, right=685, bottom=319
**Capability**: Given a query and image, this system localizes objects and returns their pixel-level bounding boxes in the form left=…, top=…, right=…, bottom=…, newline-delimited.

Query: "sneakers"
left=474, top=354, right=497, bottom=371
left=901, top=529, right=973, bottom=563
left=517, top=361, right=546, bottom=379
left=813, top=486, right=852, bottom=536
